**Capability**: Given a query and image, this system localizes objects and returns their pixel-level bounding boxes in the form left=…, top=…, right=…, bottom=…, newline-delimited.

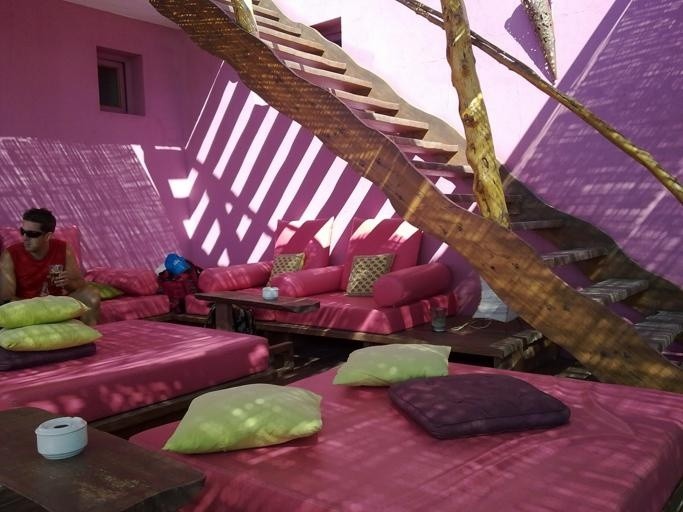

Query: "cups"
left=431, top=308, right=446, bottom=332
left=49, top=264, right=64, bottom=288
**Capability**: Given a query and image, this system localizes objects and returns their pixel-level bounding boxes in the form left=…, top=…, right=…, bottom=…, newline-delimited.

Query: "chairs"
left=197, top=216, right=457, bottom=328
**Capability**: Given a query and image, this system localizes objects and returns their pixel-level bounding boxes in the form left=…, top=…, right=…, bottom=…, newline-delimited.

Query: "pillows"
left=0, top=294, right=101, bottom=372
left=331, top=342, right=454, bottom=391
left=87, top=279, right=123, bottom=302
left=159, top=381, right=326, bottom=455
left=385, top=372, right=573, bottom=440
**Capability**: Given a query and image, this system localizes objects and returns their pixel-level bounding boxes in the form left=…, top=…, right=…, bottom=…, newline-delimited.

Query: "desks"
left=0, top=405, right=204, bottom=512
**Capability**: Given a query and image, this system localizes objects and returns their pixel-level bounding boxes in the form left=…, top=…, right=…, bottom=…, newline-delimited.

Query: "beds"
left=127, top=348, right=682, bottom=508
left=0, top=318, right=292, bottom=432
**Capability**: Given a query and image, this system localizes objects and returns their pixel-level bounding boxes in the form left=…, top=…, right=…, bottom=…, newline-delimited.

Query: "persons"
left=0, top=207, right=101, bottom=326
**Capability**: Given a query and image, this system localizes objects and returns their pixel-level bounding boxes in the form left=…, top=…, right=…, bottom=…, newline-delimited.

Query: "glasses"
left=20, top=227, right=42, bottom=238
